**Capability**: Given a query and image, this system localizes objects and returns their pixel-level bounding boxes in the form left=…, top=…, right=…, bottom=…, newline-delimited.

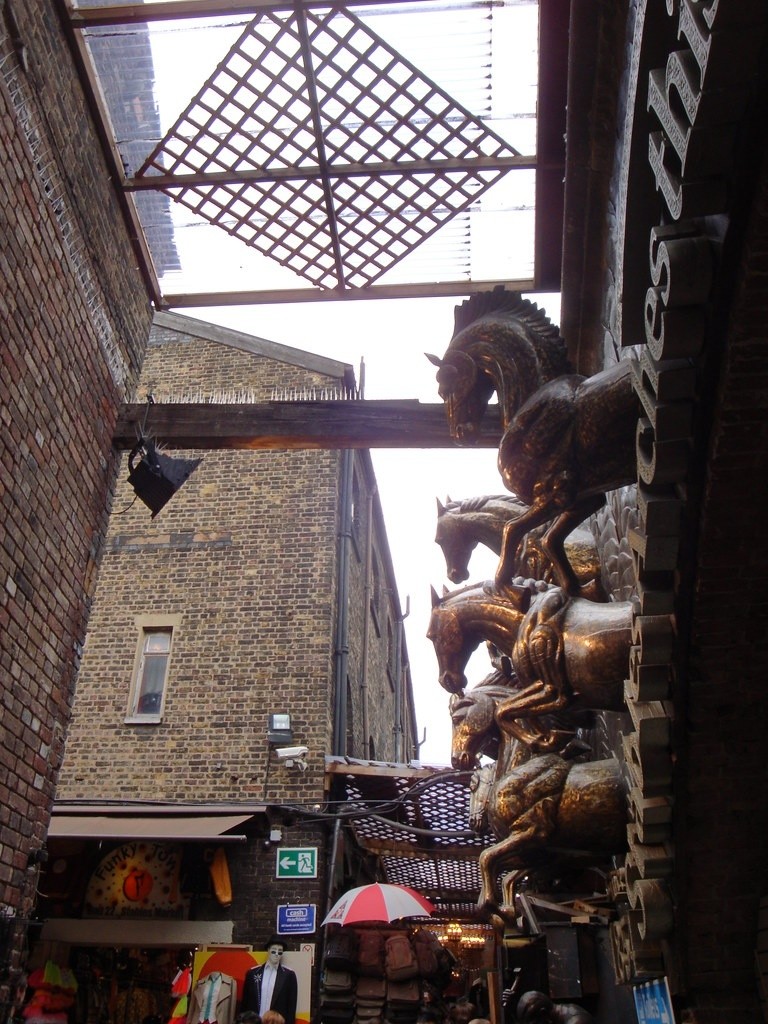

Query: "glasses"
left=269, top=950, right=283, bottom=956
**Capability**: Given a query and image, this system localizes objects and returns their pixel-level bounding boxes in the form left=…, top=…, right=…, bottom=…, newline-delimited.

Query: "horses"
left=424, top=285, right=635, bottom=920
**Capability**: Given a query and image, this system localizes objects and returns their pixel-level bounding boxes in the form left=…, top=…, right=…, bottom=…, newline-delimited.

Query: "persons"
left=502, top=988, right=597, bottom=1024
left=241, top=934, right=297, bottom=1024
left=262, top=1011, right=285, bottom=1024
left=239, top=1011, right=261, bottom=1024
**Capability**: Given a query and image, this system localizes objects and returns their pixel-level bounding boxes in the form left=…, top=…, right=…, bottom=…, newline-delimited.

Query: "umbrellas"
left=320, top=881, right=439, bottom=927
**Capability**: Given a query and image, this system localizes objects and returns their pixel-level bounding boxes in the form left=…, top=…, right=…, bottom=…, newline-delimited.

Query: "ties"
left=204, top=975, right=221, bottom=1020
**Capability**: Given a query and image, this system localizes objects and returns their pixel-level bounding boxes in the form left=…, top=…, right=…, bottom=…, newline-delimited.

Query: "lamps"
left=127, top=394, right=201, bottom=524
left=267, top=713, right=293, bottom=743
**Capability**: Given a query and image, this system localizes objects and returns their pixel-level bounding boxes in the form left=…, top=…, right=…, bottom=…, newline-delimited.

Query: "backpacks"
left=315, top=926, right=454, bottom=1024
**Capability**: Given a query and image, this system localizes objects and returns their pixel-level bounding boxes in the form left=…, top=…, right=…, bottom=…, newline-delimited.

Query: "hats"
left=264, top=934, right=288, bottom=952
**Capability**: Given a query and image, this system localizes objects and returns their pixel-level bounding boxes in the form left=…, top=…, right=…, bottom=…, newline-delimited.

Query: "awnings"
left=47, top=805, right=270, bottom=843
